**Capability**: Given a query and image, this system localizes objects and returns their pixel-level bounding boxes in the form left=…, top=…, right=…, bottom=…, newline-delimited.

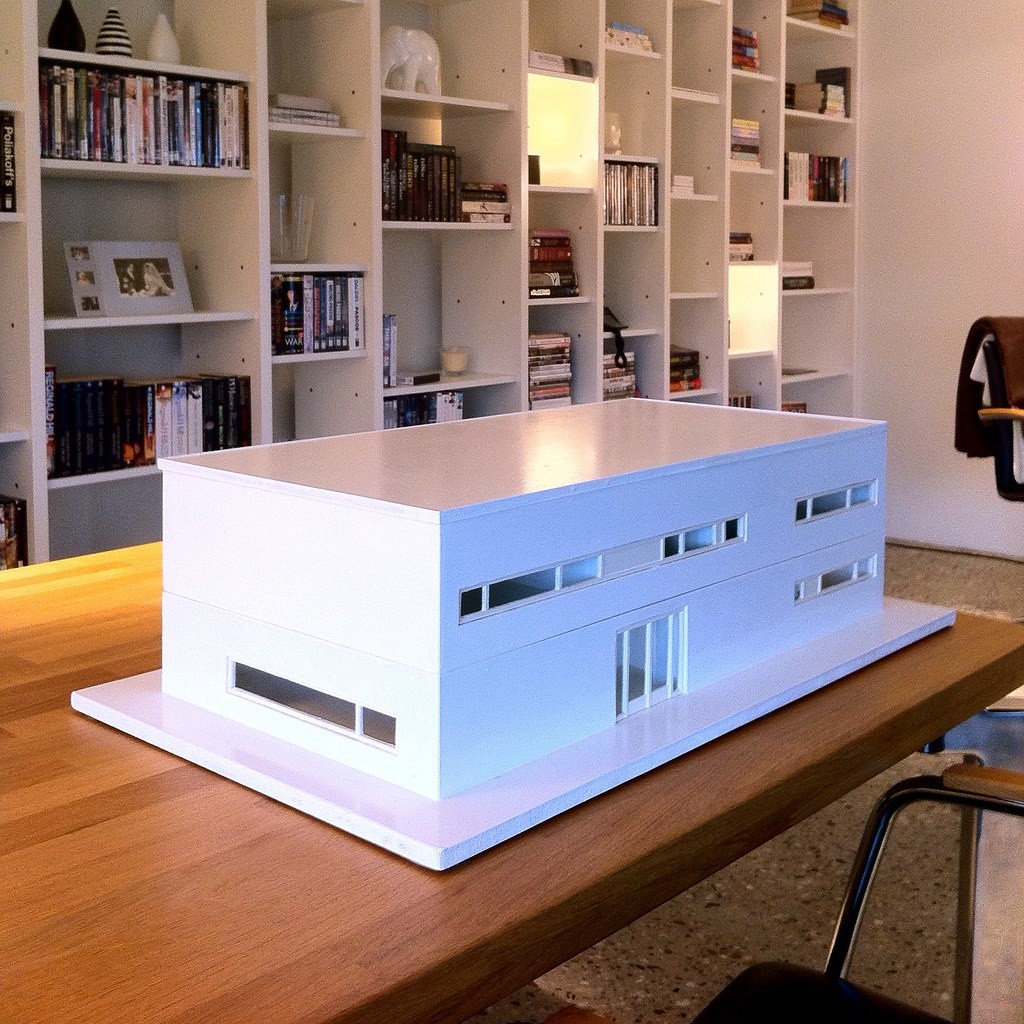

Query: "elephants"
left=380, top=25, right=440, bottom=96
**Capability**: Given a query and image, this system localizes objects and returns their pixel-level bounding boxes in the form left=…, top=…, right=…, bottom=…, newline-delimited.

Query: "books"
left=270, top=274, right=348, bottom=355
left=39, top=63, right=249, bottom=171
left=0, top=493, right=28, bottom=570
left=348, top=277, right=364, bottom=350
left=381, top=128, right=463, bottom=223
left=1, top=109, right=17, bottom=213
left=384, top=391, right=464, bottom=429
left=383, top=313, right=392, bottom=388
left=56, top=373, right=252, bottom=478
left=44, top=363, right=57, bottom=481
left=459, top=192, right=508, bottom=202
left=396, top=368, right=440, bottom=386
left=268, top=107, right=341, bottom=128
left=390, top=315, right=398, bottom=387
left=268, top=92, right=332, bottom=114
left=461, top=182, right=507, bottom=192
left=461, top=213, right=510, bottom=223
left=525, top=0, right=855, bottom=414
left=459, top=200, right=511, bottom=213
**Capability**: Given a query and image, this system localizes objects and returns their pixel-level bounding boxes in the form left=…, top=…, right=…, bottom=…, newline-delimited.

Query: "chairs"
left=954, top=316, right=1024, bottom=503
left=685, top=763, right=1024, bottom=1024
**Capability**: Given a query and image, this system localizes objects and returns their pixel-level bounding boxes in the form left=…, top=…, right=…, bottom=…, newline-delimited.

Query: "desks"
left=0, top=540, right=1024, bottom=1023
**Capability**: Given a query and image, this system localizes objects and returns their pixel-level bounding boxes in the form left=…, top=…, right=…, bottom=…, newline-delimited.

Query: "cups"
left=440, top=345, right=470, bottom=377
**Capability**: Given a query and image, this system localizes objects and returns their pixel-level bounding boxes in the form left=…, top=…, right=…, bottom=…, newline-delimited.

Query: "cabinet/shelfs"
left=1, top=1, right=861, bottom=573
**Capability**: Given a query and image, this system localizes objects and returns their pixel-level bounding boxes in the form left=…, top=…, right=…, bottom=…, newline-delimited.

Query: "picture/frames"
left=62, top=240, right=194, bottom=318
